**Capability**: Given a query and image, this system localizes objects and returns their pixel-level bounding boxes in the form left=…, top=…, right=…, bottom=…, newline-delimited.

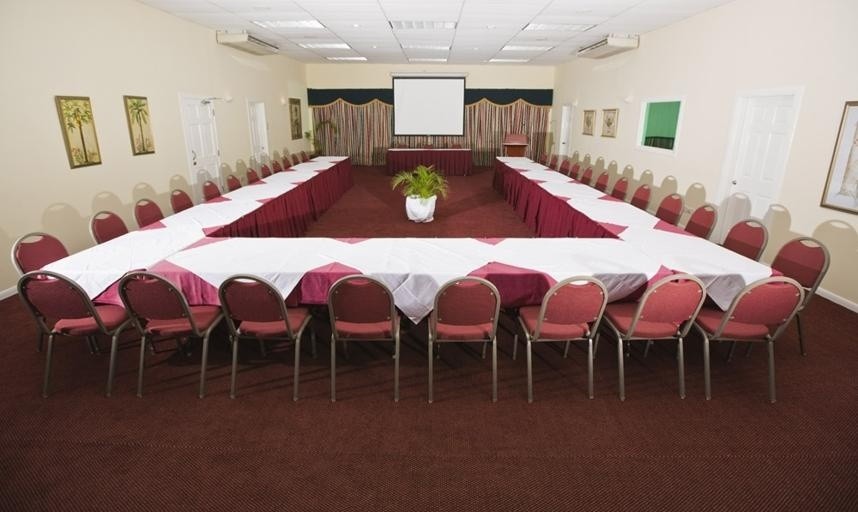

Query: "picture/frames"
left=124, top=96, right=155, bottom=155
left=583, top=109, right=596, bottom=135
left=820, top=101, right=858, bottom=215
left=55, top=95, right=101, bottom=169
left=602, top=108, right=619, bottom=138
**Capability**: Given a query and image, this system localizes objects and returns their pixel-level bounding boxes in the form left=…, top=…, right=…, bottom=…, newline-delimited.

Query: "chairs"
left=428, top=276, right=500, bottom=403
left=424, top=143, right=432, bottom=148
left=398, top=144, right=407, bottom=148
left=9, top=148, right=309, bottom=276
left=117, top=272, right=223, bottom=398
left=328, top=274, right=404, bottom=405
left=219, top=276, right=316, bottom=401
left=16, top=272, right=155, bottom=395
left=539, top=152, right=830, bottom=358
left=689, top=275, right=802, bottom=404
left=514, top=276, right=609, bottom=403
left=452, top=144, right=461, bottom=148
left=593, top=277, right=705, bottom=401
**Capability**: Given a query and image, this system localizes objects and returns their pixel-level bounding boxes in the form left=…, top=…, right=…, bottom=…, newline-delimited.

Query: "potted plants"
left=391, top=165, right=449, bottom=222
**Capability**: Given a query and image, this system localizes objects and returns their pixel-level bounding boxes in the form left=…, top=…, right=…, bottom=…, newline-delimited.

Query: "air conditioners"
left=577, top=34, right=638, bottom=60
left=217, top=30, right=279, bottom=55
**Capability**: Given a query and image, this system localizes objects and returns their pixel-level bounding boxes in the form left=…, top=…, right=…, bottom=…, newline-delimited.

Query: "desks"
left=387, top=148, right=472, bottom=175
left=20, top=236, right=780, bottom=311
left=497, top=156, right=783, bottom=300
left=126, top=154, right=351, bottom=236
